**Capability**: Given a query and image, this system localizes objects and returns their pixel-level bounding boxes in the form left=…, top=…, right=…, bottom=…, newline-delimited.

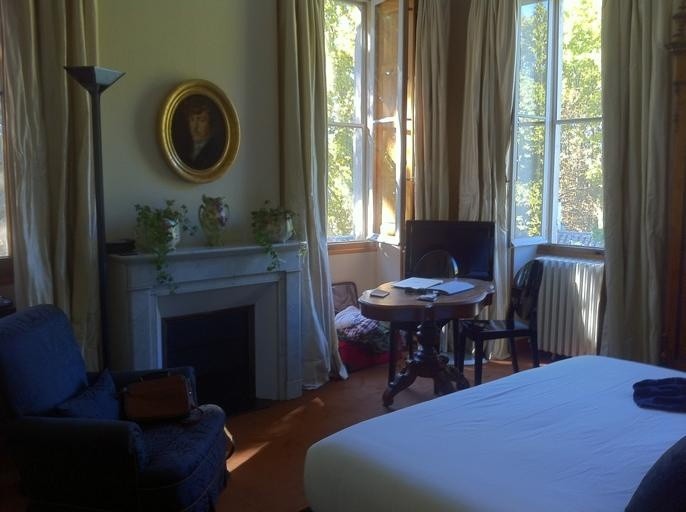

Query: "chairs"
left=453, top=258, right=547, bottom=388
left=385, top=218, right=498, bottom=387
left=0, top=304, right=232, bottom=512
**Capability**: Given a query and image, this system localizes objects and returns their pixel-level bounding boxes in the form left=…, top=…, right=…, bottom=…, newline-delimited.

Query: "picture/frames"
left=156, top=78, right=240, bottom=182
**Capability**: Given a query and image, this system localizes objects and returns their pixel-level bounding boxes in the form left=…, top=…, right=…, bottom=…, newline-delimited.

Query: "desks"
left=357, top=274, right=497, bottom=406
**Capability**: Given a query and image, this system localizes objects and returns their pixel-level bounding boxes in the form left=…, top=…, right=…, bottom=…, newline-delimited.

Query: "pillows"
left=52, top=368, right=121, bottom=426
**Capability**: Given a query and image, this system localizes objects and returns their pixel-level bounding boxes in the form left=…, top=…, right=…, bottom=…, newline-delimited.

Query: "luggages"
left=330, top=281, right=404, bottom=372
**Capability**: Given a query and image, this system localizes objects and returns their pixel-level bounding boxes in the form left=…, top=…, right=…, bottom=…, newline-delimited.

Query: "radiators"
left=533, top=255, right=606, bottom=359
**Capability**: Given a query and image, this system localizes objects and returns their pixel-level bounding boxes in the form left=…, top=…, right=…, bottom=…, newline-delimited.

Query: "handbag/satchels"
left=117, top=369, right=202, bottom=420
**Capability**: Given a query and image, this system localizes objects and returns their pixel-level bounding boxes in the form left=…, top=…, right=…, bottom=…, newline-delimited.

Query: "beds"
left=302, top=351, right=684, bottom=512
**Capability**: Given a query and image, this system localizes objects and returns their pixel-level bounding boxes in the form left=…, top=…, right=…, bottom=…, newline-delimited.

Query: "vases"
left=197, top=193, right=232, bottom=248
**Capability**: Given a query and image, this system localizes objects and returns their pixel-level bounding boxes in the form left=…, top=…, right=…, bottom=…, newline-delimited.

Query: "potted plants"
left=248, top=198, right=313, bottom=271
left=133, top=199, right=196, bottom=293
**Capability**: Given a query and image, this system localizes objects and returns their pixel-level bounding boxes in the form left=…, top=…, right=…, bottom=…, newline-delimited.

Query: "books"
left=370, top=277, right=475, bottom=301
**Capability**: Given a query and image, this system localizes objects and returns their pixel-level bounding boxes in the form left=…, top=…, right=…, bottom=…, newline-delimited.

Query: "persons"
left=182, top=98, right=217, bottom=170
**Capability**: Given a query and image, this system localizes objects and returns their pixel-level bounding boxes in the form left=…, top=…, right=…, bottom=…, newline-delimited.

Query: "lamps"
left=62, top=63, right=128, bottom=374
